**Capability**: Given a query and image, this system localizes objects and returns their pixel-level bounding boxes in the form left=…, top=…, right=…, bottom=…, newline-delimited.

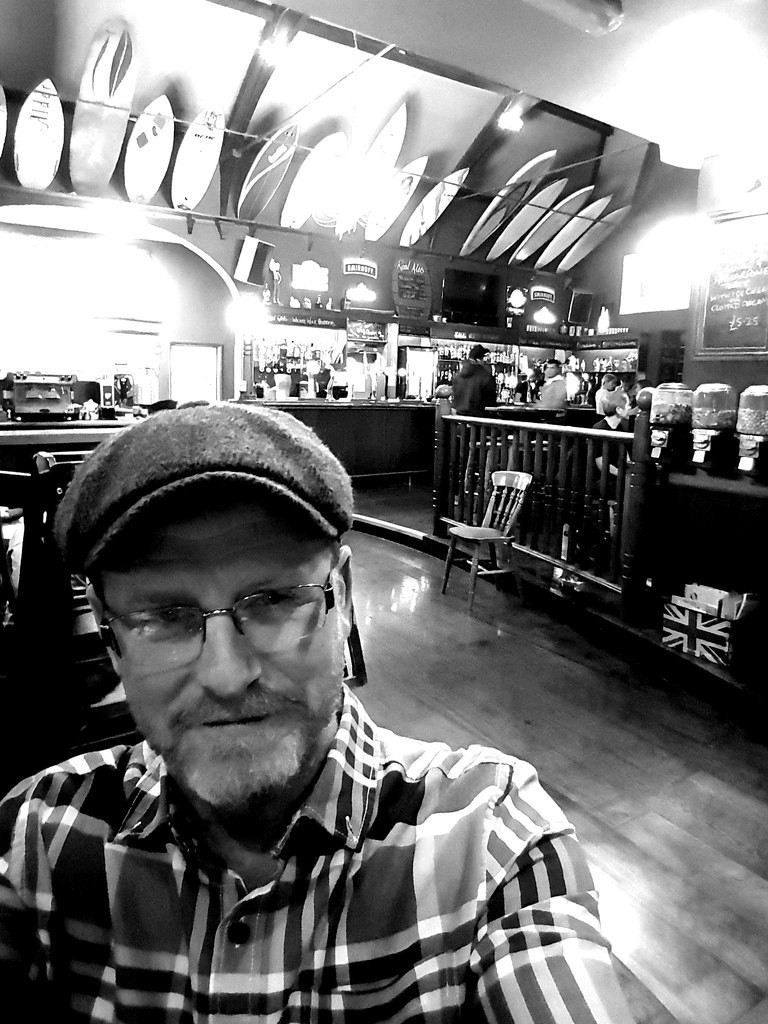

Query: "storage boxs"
left=662, top=600, right=765, bottom=666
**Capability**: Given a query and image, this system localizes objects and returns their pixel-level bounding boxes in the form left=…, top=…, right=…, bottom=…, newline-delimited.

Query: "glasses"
left=98, top=567, right=333, bottom=671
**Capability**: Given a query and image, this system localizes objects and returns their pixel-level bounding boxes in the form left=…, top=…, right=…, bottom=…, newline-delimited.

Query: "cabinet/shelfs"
left=430, top=337, right=518, bottom=401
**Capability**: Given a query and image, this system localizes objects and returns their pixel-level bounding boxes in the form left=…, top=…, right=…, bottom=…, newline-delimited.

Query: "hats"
left=469, top=345, right=490, bottom=360
left=53, top=401, right=354, bottom=572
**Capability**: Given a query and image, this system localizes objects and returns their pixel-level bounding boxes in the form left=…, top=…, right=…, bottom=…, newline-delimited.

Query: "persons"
left=451, top=344, right=654, bottom=501
left=0, top=405, right=635, bottom=1023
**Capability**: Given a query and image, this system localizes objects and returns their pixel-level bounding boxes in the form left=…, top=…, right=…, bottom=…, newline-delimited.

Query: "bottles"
left=592, top=356, right=627, bottom=372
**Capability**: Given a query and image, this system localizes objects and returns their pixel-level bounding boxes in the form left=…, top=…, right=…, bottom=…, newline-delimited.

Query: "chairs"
left=442, top=471, right=532, bottom=614
